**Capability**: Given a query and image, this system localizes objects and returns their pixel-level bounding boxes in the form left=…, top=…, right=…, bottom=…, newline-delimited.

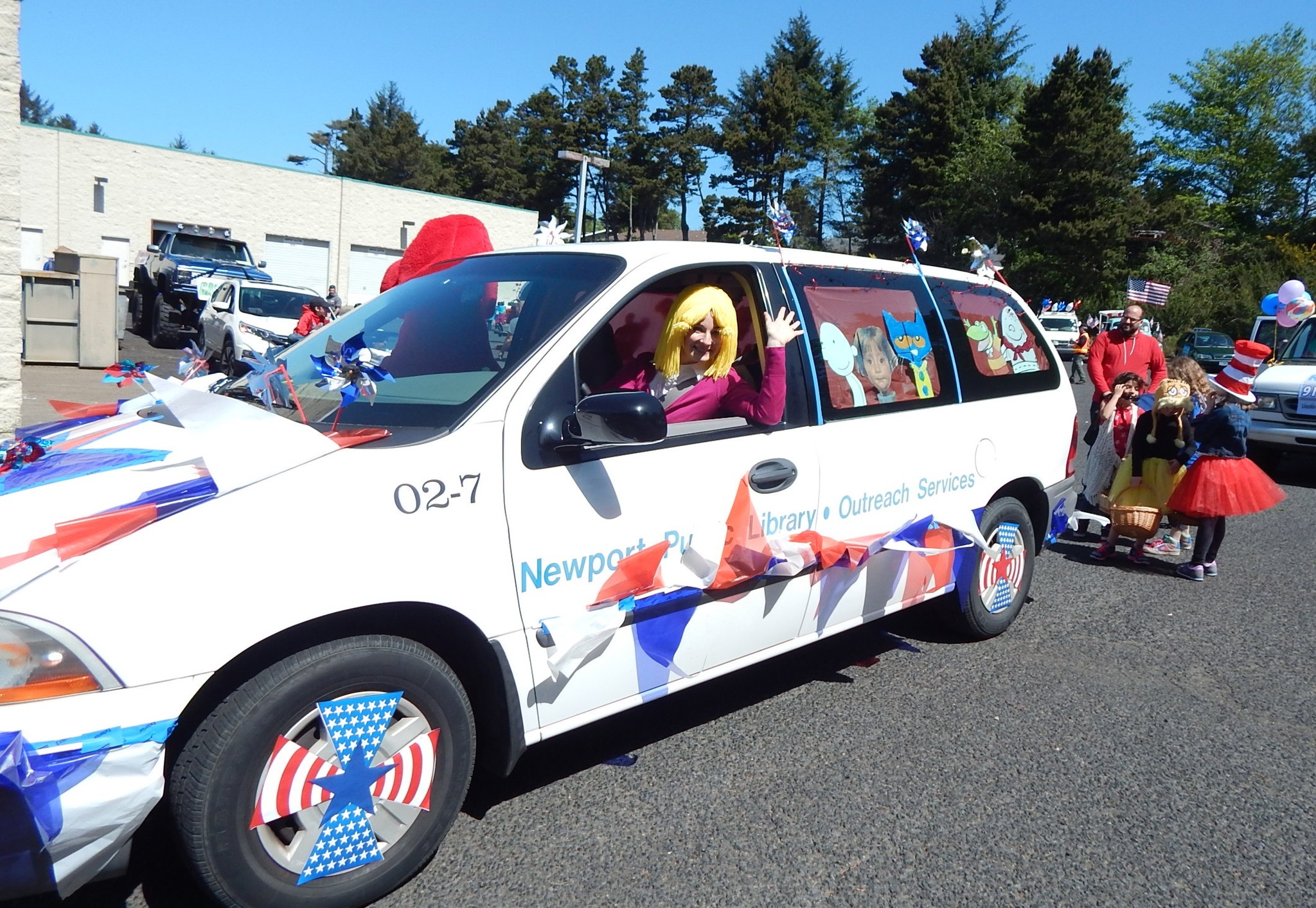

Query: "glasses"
left=1081, top=327, right=1088, bottom=331
left=1122, top=315, right=1142, bottom=322
left=1122, top=394, right=1141, bottom=402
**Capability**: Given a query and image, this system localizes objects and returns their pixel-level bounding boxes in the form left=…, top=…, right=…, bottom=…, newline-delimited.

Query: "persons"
left=1083, top=302, right=1170, bottom=448
left=1171, top=354, right=1214, bottom=430
left=587, top=283, right=807, bottom=431
left=323, top=285, right=342, bottom=317
left=1174, top=368, right=1250, bottom=581
left=1088, top=379, right=1197, bottom=566
left=286, top=297, right=333, bottom=343
left=1068, top=324, right=1090, bottom=384
left=1071, top=370, right=1148, bottom=542
left=852, top=325, right=900, bottom=403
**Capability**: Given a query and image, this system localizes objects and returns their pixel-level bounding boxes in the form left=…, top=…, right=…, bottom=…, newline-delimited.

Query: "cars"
left=1175, top=327, right=1237, bottom=372
left=2, top=237, right=1081, bottom=907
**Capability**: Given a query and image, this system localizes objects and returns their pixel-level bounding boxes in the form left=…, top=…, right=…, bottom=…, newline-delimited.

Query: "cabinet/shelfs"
left=19, top=251, right=119, bottom=370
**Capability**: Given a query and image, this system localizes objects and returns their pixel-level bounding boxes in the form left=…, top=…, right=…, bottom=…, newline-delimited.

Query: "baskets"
left=1111, top=483, right=1164, bottom=538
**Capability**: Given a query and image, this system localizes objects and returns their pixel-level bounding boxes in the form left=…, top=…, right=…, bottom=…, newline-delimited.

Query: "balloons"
left=1260, top=278, right=1315, bottom=328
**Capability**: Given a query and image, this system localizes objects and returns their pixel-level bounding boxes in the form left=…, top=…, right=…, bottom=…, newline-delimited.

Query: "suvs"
left=195, top=277, right=338, bottom=377
left=1100, top=317, right=1163, bottom=351
left=1240, top=313, right=1316, bottom=449
left=1037, top=310, right=1081, bottom=360
left=125, top=222, right=274, bottom=347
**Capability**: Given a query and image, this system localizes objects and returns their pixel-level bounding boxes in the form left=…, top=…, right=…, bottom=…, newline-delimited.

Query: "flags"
left=1126, top=277, right=1172, bottom=307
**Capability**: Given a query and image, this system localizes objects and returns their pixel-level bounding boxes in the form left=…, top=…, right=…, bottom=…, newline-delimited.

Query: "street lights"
left=557, top=149, right=611, bottom=245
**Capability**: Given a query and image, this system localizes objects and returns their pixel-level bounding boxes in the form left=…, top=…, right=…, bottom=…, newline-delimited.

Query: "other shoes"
left=1076, top=380, right=1086, bottom=384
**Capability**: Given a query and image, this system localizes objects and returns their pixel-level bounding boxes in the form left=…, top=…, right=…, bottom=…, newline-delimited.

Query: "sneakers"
left=1177, top=563, right=1204, bottom=581
left=1204, top=560, right=1217, bottom=576
left=1181, top=533, right=1191, bottom=549
left=1090, top=542, right=1116, bottom=560
left=1072, top=528, right=1086, bottom=537
left=1142, top=534, right=1181, bottom=556
left=1101, top=528, right=1109, bottom=541
left=1128, top=544, right=1150, bottom=564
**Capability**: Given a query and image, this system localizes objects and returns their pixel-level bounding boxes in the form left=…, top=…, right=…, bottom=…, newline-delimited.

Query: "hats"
left=1208, top=339, right=1272, bottom=403
left=311, top=297, right=334, bottom=308
left=1079, top=323, right=1088, bottom=327
left=1155, top=379, right=1194, bottom=415
